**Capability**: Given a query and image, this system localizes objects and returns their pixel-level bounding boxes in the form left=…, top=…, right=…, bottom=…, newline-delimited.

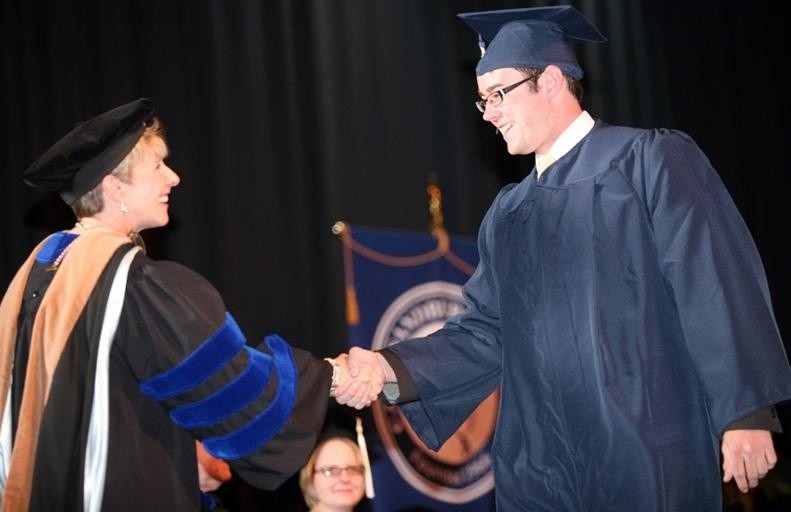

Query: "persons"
left=336, top=3, right=791, bottom=512
left=1, top=98, right=383, bottom=511
left=299, top=435, right=366, bottom=512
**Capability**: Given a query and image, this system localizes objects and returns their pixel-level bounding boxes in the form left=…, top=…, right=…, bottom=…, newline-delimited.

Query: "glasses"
left=313, top=466, right=363, bottom=477
left=475, top=76, right=533, bottom=113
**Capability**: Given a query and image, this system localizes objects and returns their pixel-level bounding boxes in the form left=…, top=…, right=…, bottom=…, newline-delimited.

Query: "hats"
left=23, top=98, right=155, bottom=206
left=457, top=5, right=608, bottom=80
